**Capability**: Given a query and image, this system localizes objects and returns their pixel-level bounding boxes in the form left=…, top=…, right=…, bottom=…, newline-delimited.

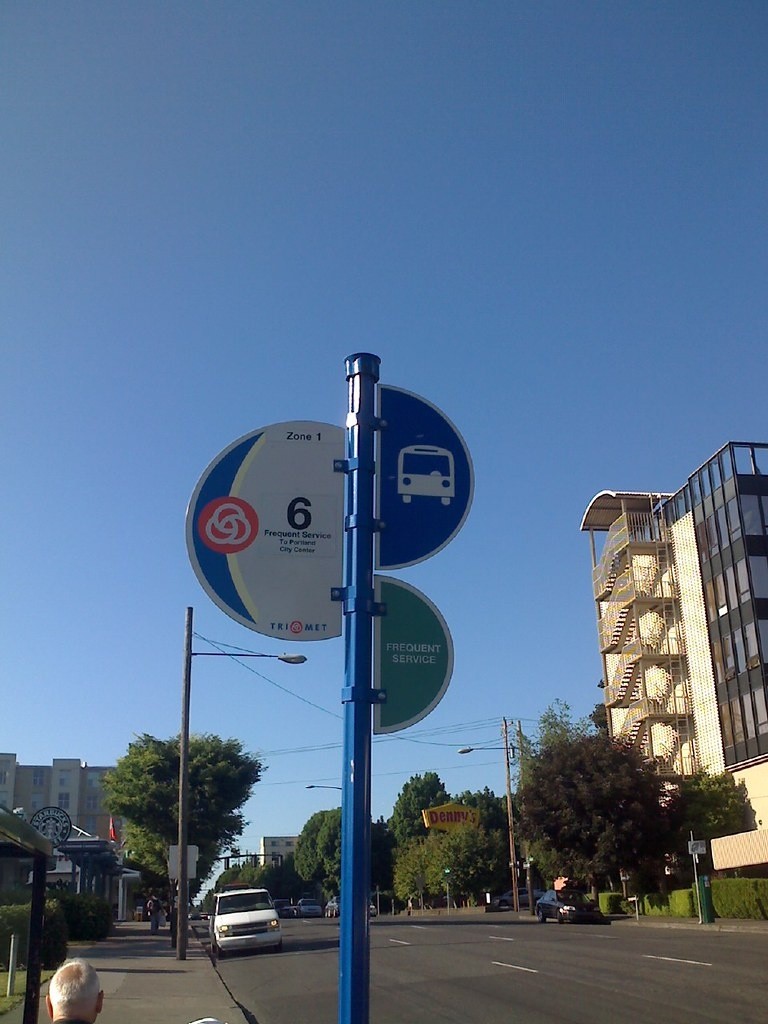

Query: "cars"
left=536, top=888, right=598, bottom=925
left=273, top=899, right=292, bottom=918
left=187, top=912, right=212, bottom=921
left=325, top=897, right=339, bottom=919
left=368, top=898, right=378, bottom=917
left=489, top=887, right=545, bottom=913
left=297, top=898, right=323, bottom=918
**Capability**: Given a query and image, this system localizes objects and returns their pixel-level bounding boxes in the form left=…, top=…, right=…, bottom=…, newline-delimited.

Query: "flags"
left=110, top=818, right=118, bottom=840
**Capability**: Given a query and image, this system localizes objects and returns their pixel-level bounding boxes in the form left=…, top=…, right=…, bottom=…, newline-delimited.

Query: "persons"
left=147, top=894, right=164, bottom=936
left=46, top=962, right=104, bottom=1024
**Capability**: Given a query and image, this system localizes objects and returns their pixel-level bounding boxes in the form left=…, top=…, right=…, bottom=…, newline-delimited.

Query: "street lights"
left=457, top=721, right=535, bottom=915
left=176, top=606, right=307, bottom=961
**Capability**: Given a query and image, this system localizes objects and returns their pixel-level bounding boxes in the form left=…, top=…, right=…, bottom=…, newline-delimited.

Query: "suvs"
left=207, top=883, right=283, bottom=961
